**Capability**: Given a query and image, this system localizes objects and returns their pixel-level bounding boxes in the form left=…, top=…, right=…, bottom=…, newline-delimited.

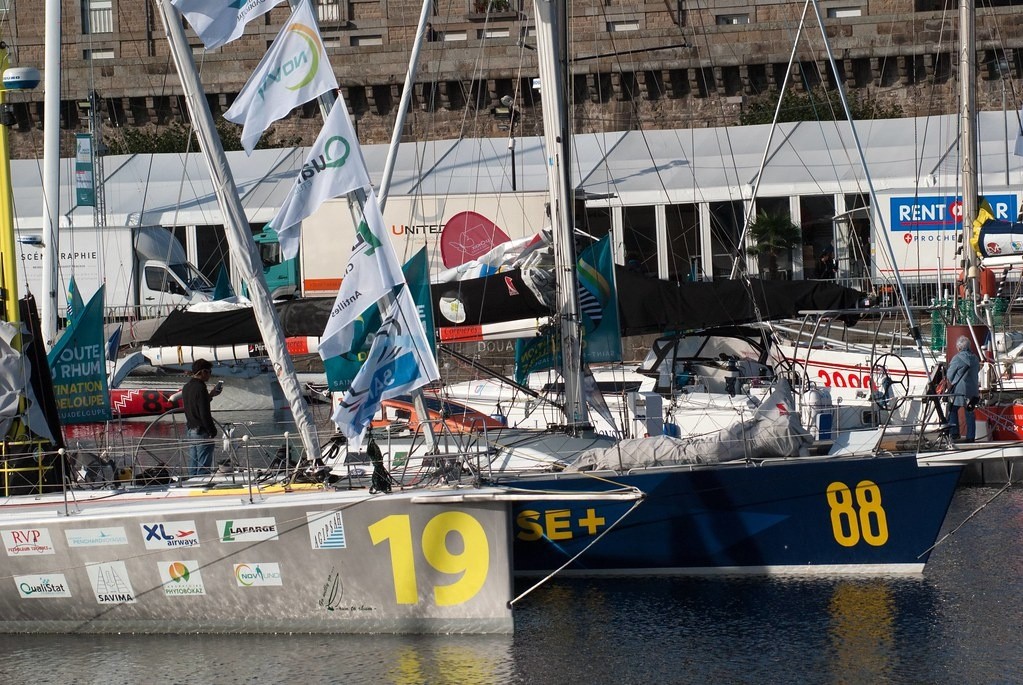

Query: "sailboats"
left=280, top=0, right=1023, bottom=579
left=4, top=0, right=640, bottom=633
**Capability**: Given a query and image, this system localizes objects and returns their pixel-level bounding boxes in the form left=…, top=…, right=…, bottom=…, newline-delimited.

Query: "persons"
left=946, top=336, right=981, bottom=442
left=820, top=247, right=837, bottom=281
left=182, top=359, right=224, bottom=479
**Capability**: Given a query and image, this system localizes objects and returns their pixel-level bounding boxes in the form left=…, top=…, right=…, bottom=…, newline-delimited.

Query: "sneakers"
left=963, top=437, right=974, bottom=443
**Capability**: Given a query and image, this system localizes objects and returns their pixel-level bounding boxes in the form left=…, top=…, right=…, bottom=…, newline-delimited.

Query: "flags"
left=319, top=191, right=440, bottom=454
left=170, top=0, right=286, bottom=50
left=269, top=90, right=371, bottom=260
left=223, top=0, right=338, bottom=157
left=516, top=233, right=621, bottom=390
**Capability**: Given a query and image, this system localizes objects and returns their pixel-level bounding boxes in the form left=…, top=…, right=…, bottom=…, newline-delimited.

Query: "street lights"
left=491, top=95, right=518, bottom=190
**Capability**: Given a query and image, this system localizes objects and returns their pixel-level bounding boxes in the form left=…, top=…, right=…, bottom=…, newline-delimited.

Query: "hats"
left=192, top=359, right=214, bottom=373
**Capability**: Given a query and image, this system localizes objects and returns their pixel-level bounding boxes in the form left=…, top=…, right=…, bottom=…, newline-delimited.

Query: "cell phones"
left=215, top=381, right=224, bottom=391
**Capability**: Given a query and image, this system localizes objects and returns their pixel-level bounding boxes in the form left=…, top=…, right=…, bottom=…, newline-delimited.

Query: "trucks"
left=870, top=188, right=1023, bottom=317
left=13, top=215, right=217, bottom=320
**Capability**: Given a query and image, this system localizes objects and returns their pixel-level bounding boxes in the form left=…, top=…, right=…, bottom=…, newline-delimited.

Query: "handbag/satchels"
left=942, top=382, right=957, bottom=401
